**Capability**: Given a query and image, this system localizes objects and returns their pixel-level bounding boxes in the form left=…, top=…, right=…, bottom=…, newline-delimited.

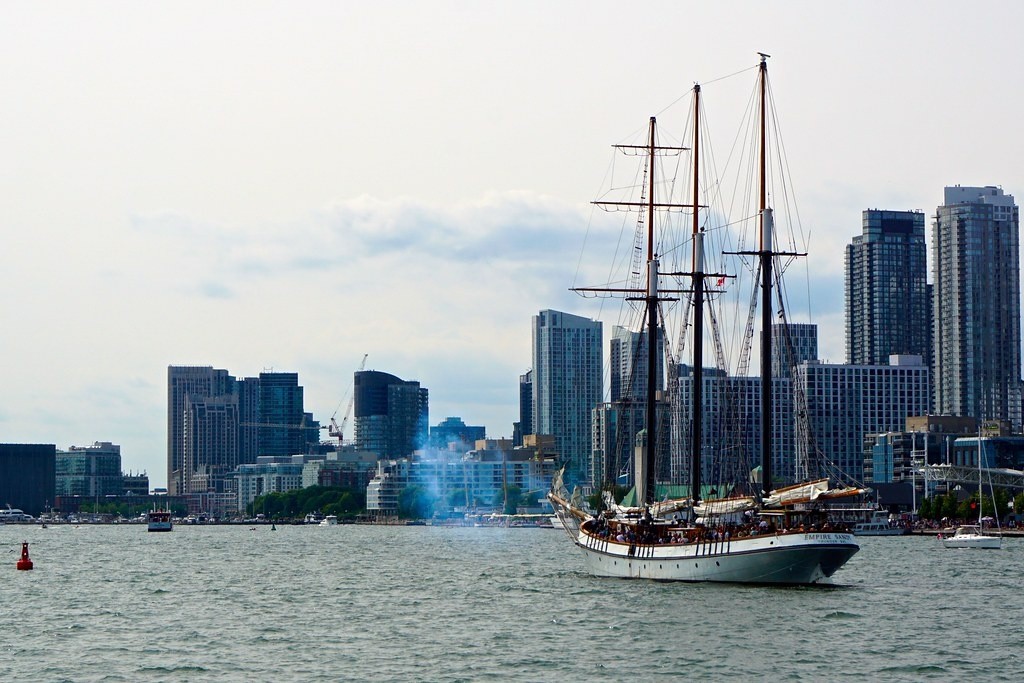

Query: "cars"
left=172, top=510, right=243, bottom=523
left=0, top=499, right=147, bottom=524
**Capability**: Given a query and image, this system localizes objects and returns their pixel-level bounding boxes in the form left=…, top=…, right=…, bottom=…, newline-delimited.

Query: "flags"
left=717, top=276, right=725, bottom=286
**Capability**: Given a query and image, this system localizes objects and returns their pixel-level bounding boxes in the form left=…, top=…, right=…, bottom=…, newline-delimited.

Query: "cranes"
left=328, top=352, right=369, bottom=453
left=240, top=414, right=330, bottom=455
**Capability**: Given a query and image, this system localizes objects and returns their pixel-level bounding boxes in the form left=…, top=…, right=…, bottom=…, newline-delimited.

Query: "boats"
left=302, top=510, right=326, bottom=524
left=320, top=514, right=338, bottom=526
left=848, top=503, right=906, bottom=536
left=147, top=501, right=173, bottom=532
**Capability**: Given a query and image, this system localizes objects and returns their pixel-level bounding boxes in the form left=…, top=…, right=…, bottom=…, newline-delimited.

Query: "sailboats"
left=941, top=424, right=1004, bottom=549
left=544, top=51, right=875, bottom=589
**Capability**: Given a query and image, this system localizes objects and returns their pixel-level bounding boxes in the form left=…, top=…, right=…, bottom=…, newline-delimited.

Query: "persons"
left=595, top=516, right=850, bottom=544
left=887, top=517, right=1024, bottom=539
